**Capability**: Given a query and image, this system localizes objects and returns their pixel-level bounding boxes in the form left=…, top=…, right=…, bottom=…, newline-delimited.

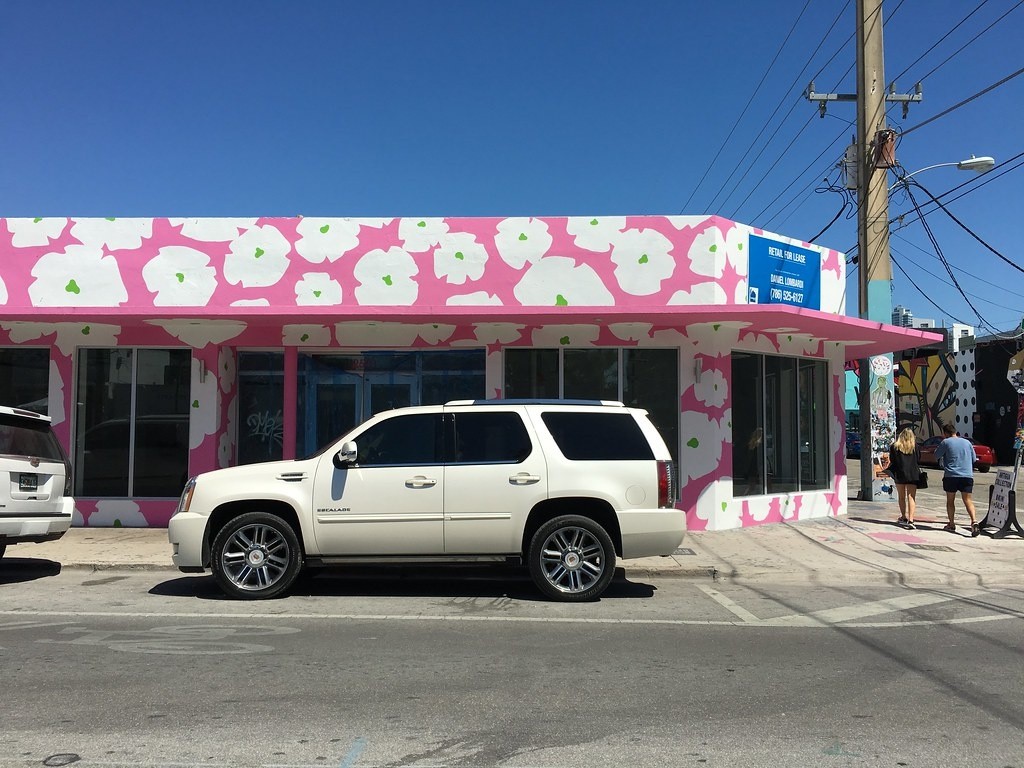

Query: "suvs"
left=0, top=405, right=76, bottom=560
left=167, top=395, right=686, bottom=601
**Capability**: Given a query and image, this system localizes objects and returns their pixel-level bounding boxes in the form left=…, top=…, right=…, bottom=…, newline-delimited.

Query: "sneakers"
left=971, top=521, right=980, bottom=537
left=944, top=522, right=955, bottom=531
left=898, top=517, right=908, bottom=525
left=908, top=521, right=916, bottom=529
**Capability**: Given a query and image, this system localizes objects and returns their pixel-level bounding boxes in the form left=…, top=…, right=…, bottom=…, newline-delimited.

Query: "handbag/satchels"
left=917, top=472, right=928, bottom=489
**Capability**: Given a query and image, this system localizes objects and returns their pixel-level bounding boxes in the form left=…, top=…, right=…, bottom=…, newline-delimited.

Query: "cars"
left=845, top=429, right=862, bottom=461
left=916, top=435, right=994, bottom=474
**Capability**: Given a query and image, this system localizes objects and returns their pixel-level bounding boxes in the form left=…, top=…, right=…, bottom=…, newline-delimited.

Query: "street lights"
left=856, top=156, right=996, bottom=505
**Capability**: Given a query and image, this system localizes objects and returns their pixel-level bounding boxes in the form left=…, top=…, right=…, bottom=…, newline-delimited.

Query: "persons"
left=889, top=428, right=921, bottom=529
left=935, top=424, right=981, bottom=537
